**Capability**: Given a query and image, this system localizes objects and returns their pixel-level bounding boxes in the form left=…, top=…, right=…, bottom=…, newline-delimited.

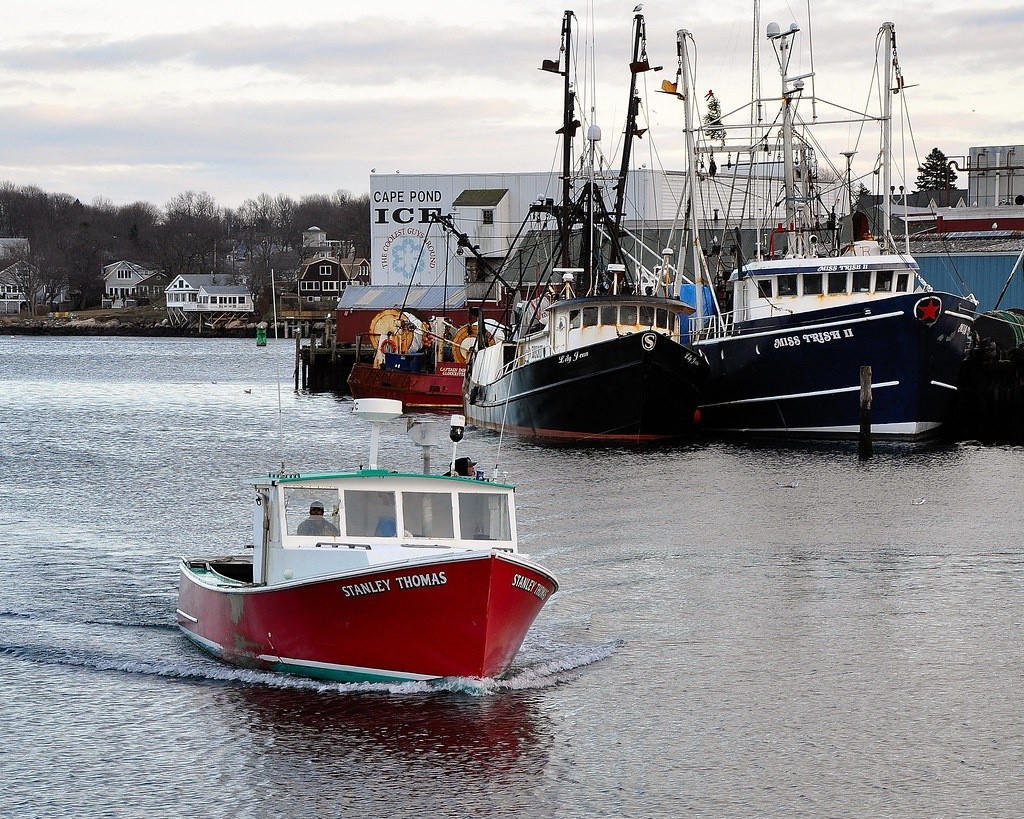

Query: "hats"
left=310, top=501, right=323, bottom=508
left=467, top=458, right=477, bottom=467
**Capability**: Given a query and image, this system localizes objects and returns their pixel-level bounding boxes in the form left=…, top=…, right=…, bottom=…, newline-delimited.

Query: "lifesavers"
left=380, top=339, right=396, bottom=354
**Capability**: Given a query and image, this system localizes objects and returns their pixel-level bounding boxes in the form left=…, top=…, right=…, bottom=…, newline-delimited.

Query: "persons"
left=297, top=501, right=339, bottom=536
left=443, top=457, right=477, bottom=477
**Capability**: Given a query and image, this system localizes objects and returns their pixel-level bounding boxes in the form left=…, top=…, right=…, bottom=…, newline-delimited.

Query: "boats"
left=351, top=3, right=980, bottom=454
left=173, top=395, right=561, bottom=686
